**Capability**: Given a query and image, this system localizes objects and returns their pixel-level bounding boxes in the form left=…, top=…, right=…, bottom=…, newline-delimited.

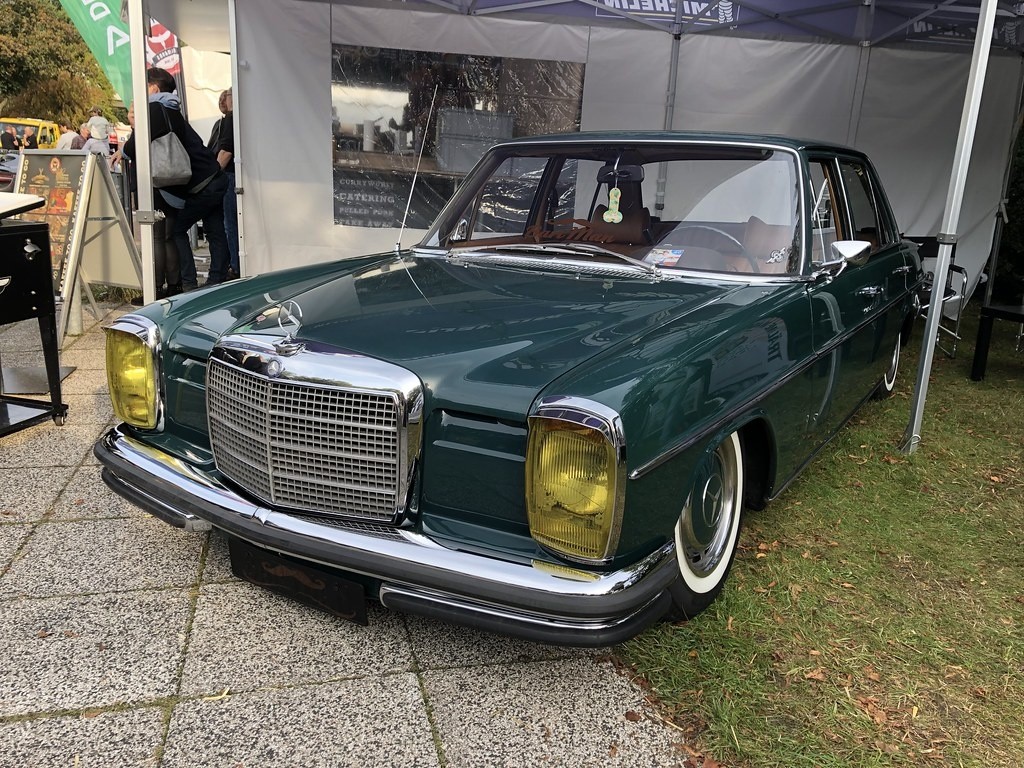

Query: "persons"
left=1, top=123, right=23, bottom=150
left=23, top=127, right=38, bottom=149
left=57, top=106, right=118, bottom=157
left=108, top=67, right=240, bottom=308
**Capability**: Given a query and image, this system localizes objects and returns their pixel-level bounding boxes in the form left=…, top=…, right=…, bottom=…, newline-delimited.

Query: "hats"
left=88, top=105, right=103, bottom=114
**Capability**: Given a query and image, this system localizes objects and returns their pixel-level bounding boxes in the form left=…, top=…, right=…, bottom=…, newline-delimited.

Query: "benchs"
left=652, top=217, right=822, bottom=260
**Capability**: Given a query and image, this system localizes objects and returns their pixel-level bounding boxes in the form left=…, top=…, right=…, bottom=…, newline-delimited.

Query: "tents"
left=120, top=1, right=1024, bottom=456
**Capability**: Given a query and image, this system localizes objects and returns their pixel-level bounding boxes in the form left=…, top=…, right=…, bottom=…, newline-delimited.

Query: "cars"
left=93, top=129, right=922, bottom=650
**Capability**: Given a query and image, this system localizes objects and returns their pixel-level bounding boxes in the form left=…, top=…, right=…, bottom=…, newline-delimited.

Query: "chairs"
left=590, top=205, right=651, bottom=245
left=743, top=215, right=787, bottom=260
left=904, top=235, right=967, bottom=359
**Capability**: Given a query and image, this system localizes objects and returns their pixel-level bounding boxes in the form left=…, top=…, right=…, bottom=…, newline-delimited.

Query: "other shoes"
left=131, top=288, right=165, bottom=306
left=200, top=281, right=214, bottom=287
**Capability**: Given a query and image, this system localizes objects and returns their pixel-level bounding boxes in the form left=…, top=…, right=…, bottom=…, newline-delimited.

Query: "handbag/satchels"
left=149, top=102, right=192, bottom=187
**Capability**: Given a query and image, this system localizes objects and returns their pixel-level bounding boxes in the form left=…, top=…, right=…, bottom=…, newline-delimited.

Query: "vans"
left=0, top=118, right=59, bottom=152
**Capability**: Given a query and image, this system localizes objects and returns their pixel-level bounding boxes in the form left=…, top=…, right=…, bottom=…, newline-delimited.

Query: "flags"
left=59, top=0, right=133, bottom=112
left=145, top=18, right=181, bottom=76
